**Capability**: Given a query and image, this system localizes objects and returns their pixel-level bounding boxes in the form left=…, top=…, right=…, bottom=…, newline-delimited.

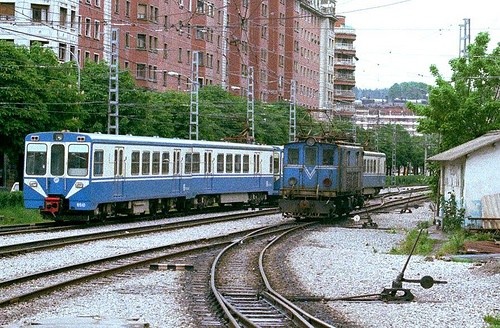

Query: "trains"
left=25, top=131, right=387, bottom=223
left=275, top=135, right=365, bottom=223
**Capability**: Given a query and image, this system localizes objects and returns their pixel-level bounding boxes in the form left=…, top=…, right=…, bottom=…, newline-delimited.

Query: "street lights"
left=167, top=70, right=199, bottom=140
left=232, top=85, right=255, bottom=144
left=40, top=43, right=81, bottom=96
